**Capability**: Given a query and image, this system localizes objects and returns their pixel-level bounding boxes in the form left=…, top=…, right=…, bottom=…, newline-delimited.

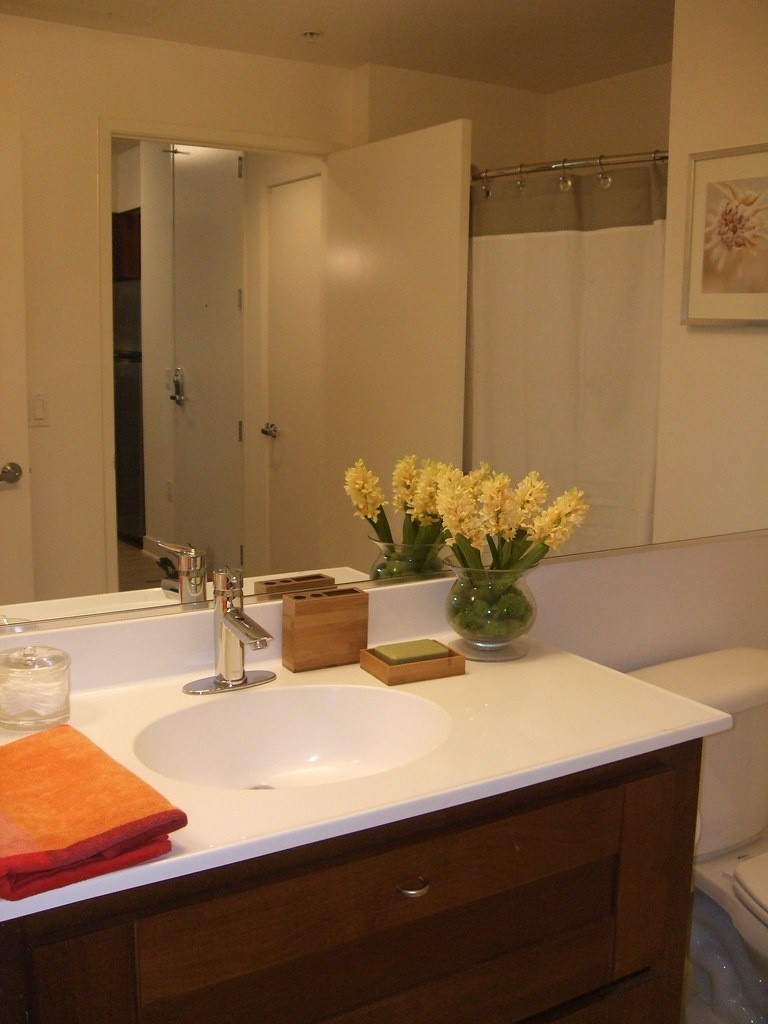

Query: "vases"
left=365, top=529, right=453, bottom=580
left=442, top=550, right=545, bottom=664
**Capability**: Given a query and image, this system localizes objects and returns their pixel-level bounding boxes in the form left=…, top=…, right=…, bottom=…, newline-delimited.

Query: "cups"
left=0, top=645, right=71, bottom=732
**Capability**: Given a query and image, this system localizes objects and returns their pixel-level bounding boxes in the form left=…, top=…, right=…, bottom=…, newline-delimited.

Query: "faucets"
left=141, top=535, right=207, bottom=603
left=212, top=565, right=275, bottom=686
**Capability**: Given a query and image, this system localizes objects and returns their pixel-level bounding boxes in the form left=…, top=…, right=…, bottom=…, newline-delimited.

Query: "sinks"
left=134, top=685, right=454, bottom=791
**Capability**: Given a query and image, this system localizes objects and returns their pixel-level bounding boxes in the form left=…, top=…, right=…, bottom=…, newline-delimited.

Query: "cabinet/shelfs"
left=0, top=737, right=705, bottom=1024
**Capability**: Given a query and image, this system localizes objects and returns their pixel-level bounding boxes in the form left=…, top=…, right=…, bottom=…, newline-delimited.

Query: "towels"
left=0, top=724, right=188, bottom=901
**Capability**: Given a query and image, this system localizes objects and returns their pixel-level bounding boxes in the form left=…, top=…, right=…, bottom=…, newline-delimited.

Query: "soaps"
left=373, top=639, right=449, bottom=665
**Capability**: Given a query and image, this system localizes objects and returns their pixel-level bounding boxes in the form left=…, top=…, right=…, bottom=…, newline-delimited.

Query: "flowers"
left=0, top=0, right=674, bottom=636
left=435, top=461, right=591, bottom=570
left=343, top=453, right=466, bottom=545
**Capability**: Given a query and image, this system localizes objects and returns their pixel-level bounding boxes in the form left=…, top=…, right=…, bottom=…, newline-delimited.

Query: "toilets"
left=625, top=645, right=768, bottom=1024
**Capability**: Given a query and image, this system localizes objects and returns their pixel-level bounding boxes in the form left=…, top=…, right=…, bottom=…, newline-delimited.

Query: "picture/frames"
left=679, top=140, right=768, bottom=328
